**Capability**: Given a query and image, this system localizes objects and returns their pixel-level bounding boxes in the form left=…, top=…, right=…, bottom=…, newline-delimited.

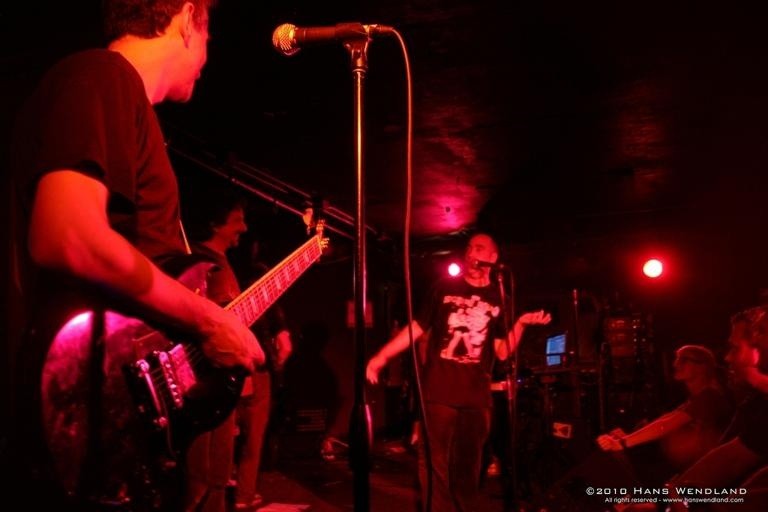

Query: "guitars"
left=41, top=219, right=330, bottom=512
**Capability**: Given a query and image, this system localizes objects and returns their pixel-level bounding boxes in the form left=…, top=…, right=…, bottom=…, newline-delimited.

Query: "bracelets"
left=618, top=437, right=630, bottom=451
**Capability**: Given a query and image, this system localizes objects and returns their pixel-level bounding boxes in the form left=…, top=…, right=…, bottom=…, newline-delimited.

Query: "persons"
left=234, top=236, right=295, bottom=512
left=2, top=0, right=267, bottom=511
left=187, top=183, right=266, bottom=512
left=612, top=303, right=768, bottom=512
left=365, top=232, right=552, bottom=512
left=596, top=344, right=738, bottom=468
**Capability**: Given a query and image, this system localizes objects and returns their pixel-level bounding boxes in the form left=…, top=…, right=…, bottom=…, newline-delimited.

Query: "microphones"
left=272, top=23, right=394, bottom=56
left=471, top=259, right=510, bottom=271
